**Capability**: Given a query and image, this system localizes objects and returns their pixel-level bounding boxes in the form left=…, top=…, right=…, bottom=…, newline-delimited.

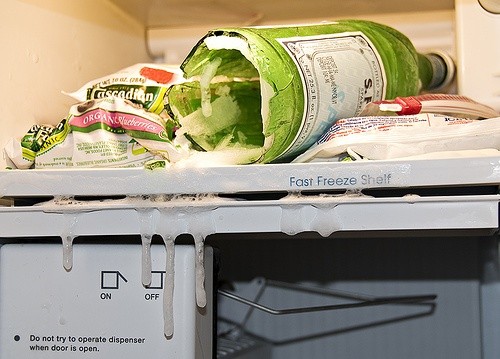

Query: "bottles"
left=162, top=17, right=457, bottom=165
